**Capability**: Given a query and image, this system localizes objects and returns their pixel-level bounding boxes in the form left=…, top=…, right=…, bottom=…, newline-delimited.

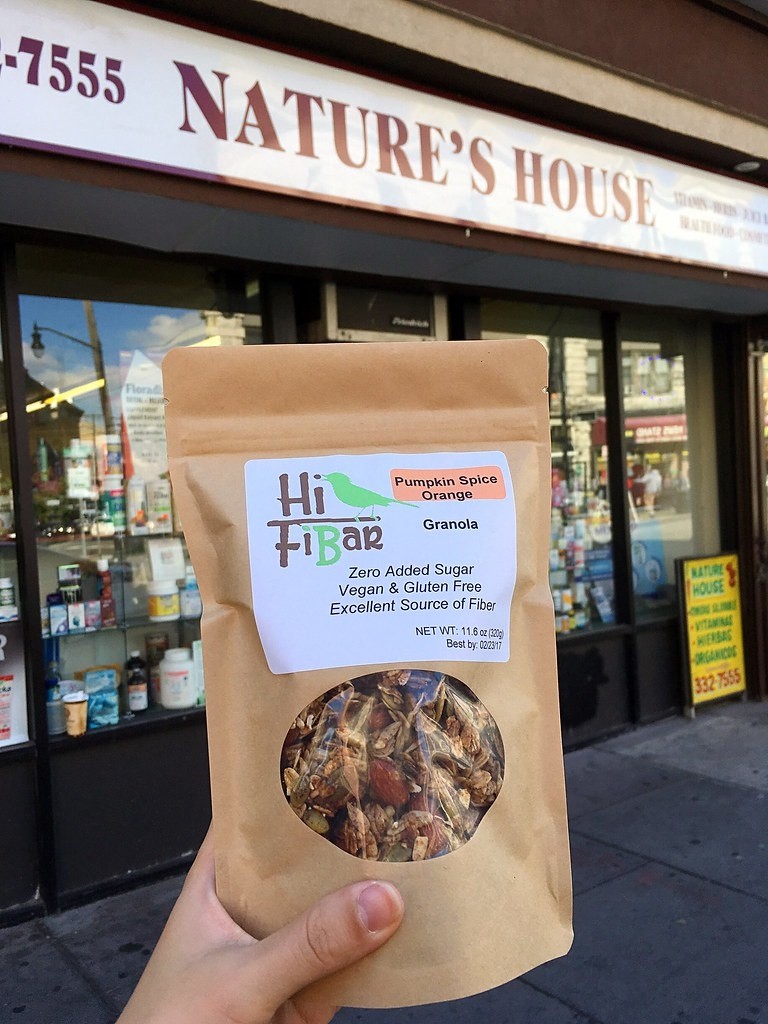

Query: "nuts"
left=281, top=669, right=506, bottom=863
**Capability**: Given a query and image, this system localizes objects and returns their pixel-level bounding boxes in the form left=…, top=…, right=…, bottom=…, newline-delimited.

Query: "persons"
left=633, top=464, right=664, bottom=517
left=110, top=813, right=405, bottom=1023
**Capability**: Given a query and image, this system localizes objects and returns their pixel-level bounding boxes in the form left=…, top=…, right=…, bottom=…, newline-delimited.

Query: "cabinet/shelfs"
left=0, top=346, right=37, bottom=753
left=622, top=339, right=700, bottom=614
left=547, top=353, right=620, bottom=638
left=36, top=353, right=206, bottom=742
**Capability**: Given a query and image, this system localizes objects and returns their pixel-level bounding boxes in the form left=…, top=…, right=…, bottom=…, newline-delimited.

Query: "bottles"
left=0, top=434, right=207, bottom=737
left=547, top=471, right=613, bottom=637
